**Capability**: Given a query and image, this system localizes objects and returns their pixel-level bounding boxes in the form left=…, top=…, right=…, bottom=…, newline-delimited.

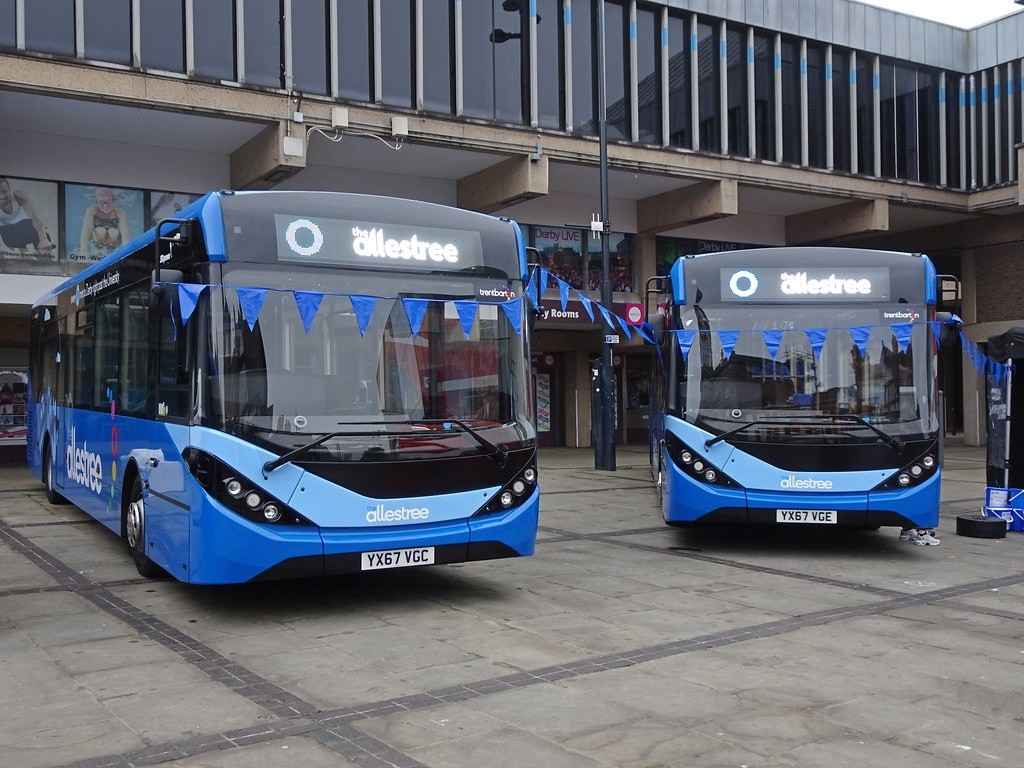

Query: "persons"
left=151, top=193, right=193, bottom=228
left=80, top=187, right=129, bottom=263
left=542, top=266, right=633, bottom=292
left=1, top=178, right=53, bottom=255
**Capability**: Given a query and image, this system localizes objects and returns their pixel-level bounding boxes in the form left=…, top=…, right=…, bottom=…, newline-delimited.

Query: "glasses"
left=97, top=198, right=115, bottom=207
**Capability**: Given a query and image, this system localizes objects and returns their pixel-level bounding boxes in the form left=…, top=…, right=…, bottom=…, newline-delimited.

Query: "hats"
left=94, top=187, right=113, bottom=199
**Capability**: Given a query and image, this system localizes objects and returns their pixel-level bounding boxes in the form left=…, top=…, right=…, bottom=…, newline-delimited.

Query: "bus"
left=26, top=189, right=550, bottom=587
left=643, top=246, right=960, bottom=532
